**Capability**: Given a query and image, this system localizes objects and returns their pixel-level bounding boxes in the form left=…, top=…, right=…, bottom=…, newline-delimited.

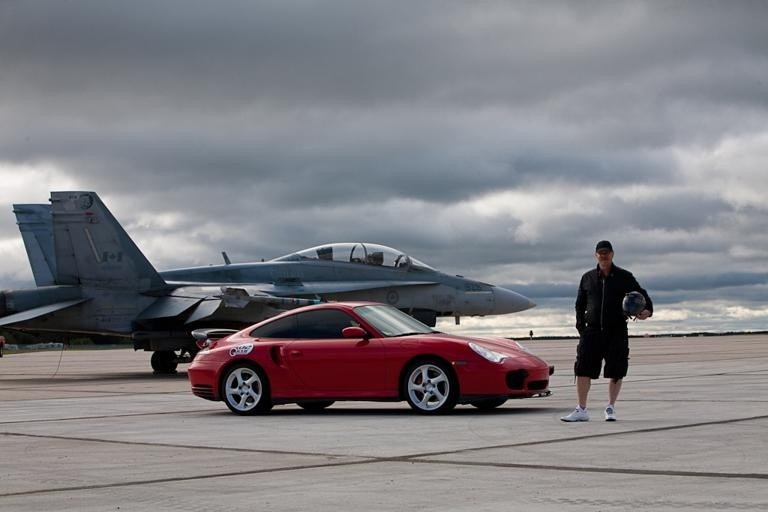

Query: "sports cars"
left=186, top=297, right=557, bottom=420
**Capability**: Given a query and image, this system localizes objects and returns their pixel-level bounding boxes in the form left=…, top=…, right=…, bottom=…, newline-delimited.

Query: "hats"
left=596, top=240, right=614, bottom=254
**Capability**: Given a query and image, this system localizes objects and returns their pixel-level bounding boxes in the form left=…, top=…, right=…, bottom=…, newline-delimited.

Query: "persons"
left=560, top=240, right=652, bottom=421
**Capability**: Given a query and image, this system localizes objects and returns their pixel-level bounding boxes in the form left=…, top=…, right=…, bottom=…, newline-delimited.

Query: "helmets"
left=624, top=290, right=646, bottom=321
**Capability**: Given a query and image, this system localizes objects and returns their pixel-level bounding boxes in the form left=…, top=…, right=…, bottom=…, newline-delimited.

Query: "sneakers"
left=560, top=406, right=590, bottom=421
left=604, top=406, right=616, bottom=421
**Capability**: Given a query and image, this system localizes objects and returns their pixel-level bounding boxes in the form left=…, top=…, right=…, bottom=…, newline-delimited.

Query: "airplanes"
left=0, top=186, right=541, bottom=381
